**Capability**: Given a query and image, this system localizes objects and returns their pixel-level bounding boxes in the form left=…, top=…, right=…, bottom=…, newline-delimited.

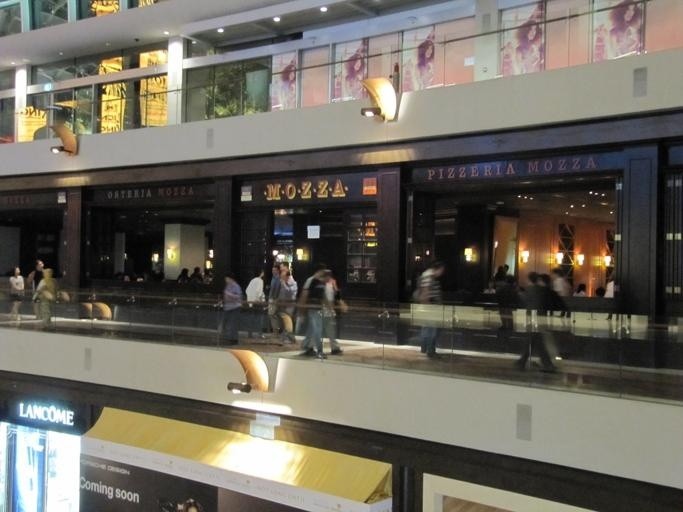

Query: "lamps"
left=577, top=254, right=584, bottom=265
left=296, top=249, right=304, bottom=260
left=464, top=247, right=474, bottom=263
left=555, top=252, right=563, bottom=264
left=359, top=77, right=396, bottom=122
left=605, top=256, right=612, bottom=266
left=48, top=125, right=78, bottom=156
left=227, top=350, right=269, bottom=394
left=522, top=251, right=530, bottom=263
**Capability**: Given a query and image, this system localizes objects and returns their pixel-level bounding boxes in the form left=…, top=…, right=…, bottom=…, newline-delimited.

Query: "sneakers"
left=304, top=350, right=326, bottom=359
left=331, top=349, right=342, bottom=355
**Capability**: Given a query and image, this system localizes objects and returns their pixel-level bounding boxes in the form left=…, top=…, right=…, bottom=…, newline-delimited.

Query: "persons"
left=281, top=41, right=434, bottom=112
left=176, top=267, right=204, bottom=283
left=218, top=260, right=344, bottom=357
left=9, top=260, right=58, bottom=331
left=496, top=1, right=641, bottom=76
left=413, top=262, right=613, bottom=372
